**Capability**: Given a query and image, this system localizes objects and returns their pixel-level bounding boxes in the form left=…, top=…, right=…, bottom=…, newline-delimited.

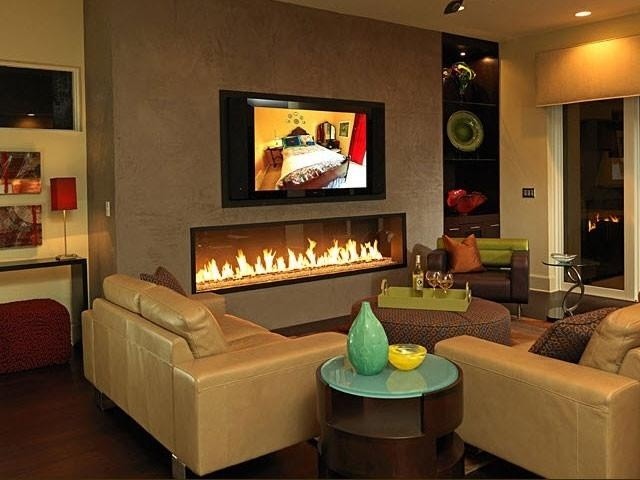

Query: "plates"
left=447, top=111, right=484, bottom=151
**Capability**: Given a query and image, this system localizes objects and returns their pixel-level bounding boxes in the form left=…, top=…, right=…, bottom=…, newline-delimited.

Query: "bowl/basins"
left=386, top=368, right=428, bottom=392
left=387, top=344, right=426, bottom=371
left=550, top=253, right=577, bottom=263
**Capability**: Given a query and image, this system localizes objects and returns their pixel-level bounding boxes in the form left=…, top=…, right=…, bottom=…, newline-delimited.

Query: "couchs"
left=79, top=291, right=346, bottom=480
left=431, top=302, right=640, bottom=480
left=427, top=235, right=532, bottom=321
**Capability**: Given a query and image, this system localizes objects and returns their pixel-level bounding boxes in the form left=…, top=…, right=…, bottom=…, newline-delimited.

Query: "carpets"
left=284, top=314, right=556, bottom=477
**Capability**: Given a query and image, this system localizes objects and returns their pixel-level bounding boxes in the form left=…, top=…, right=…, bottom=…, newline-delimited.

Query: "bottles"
left=411, top=255, right=425, bottom=297
left=347, top=300, right=388, bottom=374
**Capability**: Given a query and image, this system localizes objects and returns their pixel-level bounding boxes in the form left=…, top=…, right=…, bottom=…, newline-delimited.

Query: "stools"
left=0, top=298, right=71, bottom=374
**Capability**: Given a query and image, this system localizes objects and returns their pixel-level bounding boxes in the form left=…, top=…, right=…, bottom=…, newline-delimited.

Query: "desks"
left=1, top=255, right=93, bottom=362
left=541, top=254, right=599, bottom=322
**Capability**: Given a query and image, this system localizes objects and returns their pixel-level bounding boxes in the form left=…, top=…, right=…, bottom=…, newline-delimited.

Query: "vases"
left=346, top=300, right=387, bottom=376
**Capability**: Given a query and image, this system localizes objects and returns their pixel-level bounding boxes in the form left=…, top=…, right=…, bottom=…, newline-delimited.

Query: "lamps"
left=49, top=176, right=77, bottom=260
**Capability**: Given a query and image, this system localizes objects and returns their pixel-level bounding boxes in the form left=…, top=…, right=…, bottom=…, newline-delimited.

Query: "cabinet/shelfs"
left=314, top=350, right=463, bottom=480
left=443, top=30, right=501, bottom=237
left=582, top=219, right=622, bottom=279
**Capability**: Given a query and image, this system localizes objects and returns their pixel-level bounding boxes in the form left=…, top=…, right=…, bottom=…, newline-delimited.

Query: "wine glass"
left=426, top=271, right=441, bottom=298
left=436, top=273, right=454, bottom=298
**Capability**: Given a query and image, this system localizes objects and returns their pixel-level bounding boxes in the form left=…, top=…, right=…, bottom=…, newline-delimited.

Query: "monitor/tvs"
left=227, top=92, right=373, bottom=200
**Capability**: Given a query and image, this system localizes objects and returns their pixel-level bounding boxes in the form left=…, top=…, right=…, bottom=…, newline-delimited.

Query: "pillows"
left=100, top=267, right=153, bottom=313
left=137, top=266, right=184, bottom=297
left=529, top=305, right=616, bottom=364
left=133, top=287, right=223, bottom=358
left=444, top=233, right=484, bottom=275
